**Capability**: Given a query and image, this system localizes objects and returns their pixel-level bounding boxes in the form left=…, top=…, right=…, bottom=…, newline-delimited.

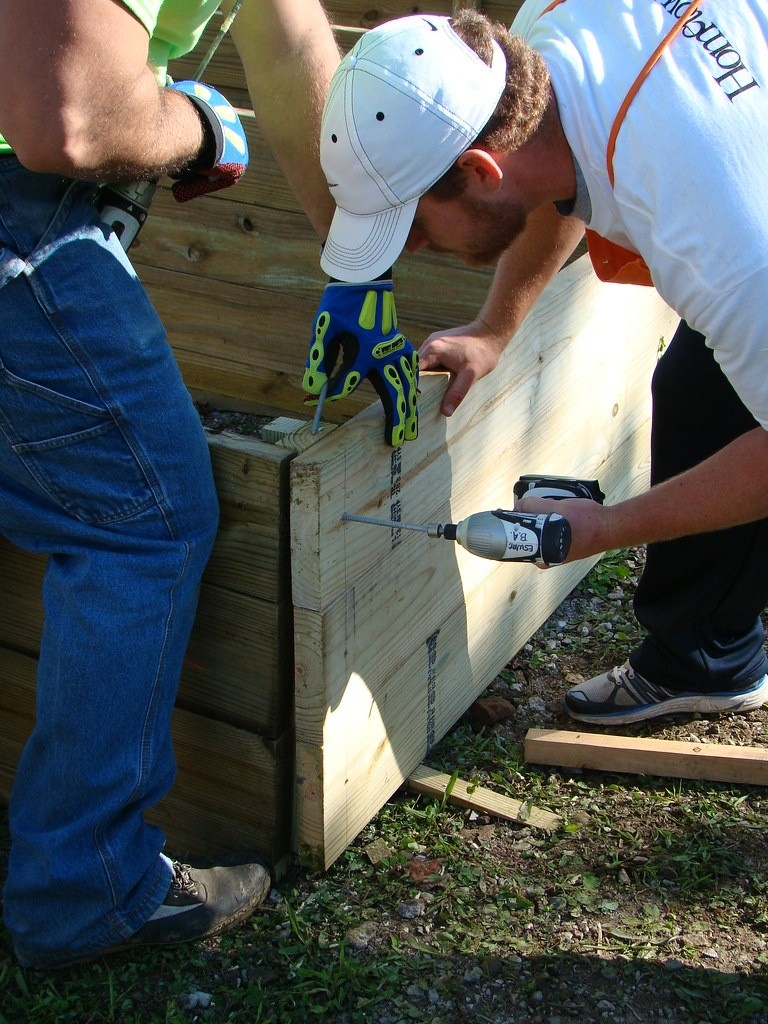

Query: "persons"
left=0, top=0, right=423, bottom=970
left=317, top=0, right=767, bottom=728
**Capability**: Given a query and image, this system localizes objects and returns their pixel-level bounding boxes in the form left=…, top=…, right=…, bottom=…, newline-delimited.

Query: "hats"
left=320, top=13, right=510, bottom=282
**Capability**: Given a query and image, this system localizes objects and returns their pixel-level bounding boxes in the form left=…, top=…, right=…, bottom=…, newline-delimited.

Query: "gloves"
left=301, top=241, right=421, bottom=447
left=167, top=77, right=251, bottom=202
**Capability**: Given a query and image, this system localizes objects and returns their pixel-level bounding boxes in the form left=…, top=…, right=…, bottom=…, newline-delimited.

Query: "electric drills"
left=97, top=0, right=243, bottom=255
left=340, top=472, right=606, bottom=571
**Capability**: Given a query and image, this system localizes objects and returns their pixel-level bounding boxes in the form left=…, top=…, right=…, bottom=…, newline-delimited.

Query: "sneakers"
left=565, top=659, right=768, bottom=725
left=15, top=851, right=271, bottom=971
